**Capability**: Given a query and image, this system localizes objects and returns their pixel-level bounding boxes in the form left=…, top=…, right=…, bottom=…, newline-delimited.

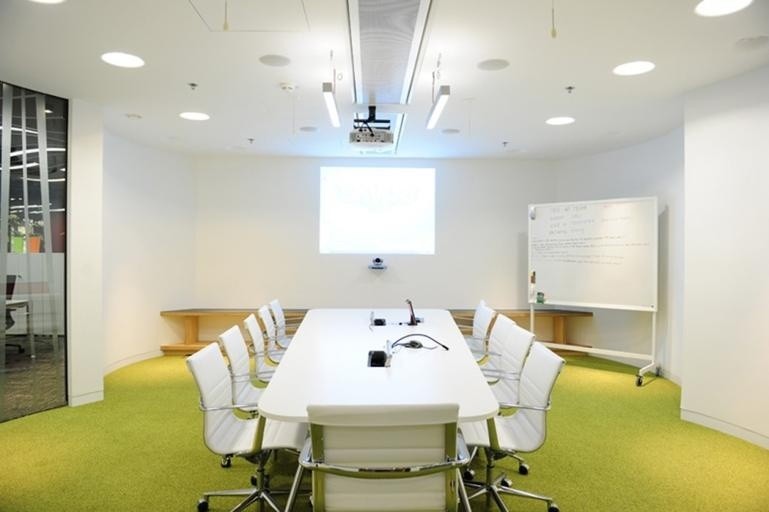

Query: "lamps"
left=321, top=51, right=342, bottom=129
left=424, top=56, right=451, bottom=131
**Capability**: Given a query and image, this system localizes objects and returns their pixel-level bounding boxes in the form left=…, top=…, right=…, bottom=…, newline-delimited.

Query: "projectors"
left=348, top=129, right=393, bottom=150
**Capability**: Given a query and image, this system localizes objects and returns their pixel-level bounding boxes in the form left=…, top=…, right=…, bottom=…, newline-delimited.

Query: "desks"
left=157, top=306, right=595, bottom=358
left=2, top=299, right=37, bottom=366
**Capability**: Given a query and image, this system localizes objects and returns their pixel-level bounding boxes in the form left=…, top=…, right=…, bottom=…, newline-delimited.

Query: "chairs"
left=3, top=273, right=25, bottom=355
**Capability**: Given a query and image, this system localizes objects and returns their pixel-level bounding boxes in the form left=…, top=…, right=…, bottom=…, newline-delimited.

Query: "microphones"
left=405, top=296, right=417, bottom=325
left=392, top=334, right=448, bottom=350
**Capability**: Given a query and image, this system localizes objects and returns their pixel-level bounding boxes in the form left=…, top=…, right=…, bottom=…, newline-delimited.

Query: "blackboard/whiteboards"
left=527, top=195, right=658, bottom=312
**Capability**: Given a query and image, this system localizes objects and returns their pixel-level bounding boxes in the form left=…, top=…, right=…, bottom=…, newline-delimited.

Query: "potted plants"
left=1, top=214, right=45, bottom=254
